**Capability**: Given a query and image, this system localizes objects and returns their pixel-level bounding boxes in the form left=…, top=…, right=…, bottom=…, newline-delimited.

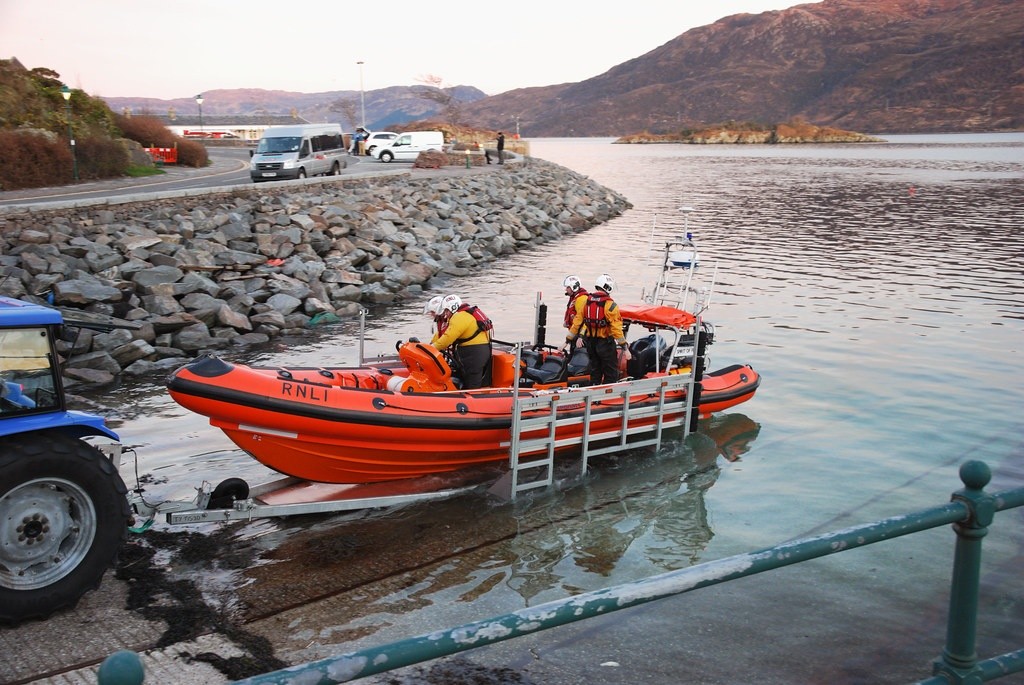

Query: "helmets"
left=594, top=273, right=616, bottom=295
left=422, top=294, right=444, bottom=315
left=564, top=274, right=582, bottom=293
left=441, top=293, right=463, bottom=313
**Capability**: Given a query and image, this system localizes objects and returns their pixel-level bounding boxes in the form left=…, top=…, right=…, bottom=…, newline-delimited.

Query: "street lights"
left=59, top=86, right=78, bottom=182
left=356, top=61, right=365, bottom=127
left=195, top=94, right=205, bottom=147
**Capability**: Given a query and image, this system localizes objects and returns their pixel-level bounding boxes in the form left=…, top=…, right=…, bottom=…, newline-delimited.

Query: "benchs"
left=521, top=344, right=567, bottom=383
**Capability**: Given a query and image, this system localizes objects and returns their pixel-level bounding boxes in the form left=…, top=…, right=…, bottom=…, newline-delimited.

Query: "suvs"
left=351, top=126, right=402, bottom=157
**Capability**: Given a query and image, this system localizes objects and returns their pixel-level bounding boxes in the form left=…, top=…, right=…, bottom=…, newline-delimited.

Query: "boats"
left=167, top=207, right=761, bottom=484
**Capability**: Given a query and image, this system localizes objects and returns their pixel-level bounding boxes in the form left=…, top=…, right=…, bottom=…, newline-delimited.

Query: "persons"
left=423, top=294, right=492, bottom=390
left=353, top=130, right=365, bottom=155
left=496, top=132, right=505, bottom=165
left=563, top=275, right=589, bottom=366
left=558, top=274, right=631, bottom=385
left=479, top=144, right=493, bottom=164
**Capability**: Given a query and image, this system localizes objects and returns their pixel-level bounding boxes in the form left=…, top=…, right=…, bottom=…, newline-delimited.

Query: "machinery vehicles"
left=0, top=296, right=135, bottom=628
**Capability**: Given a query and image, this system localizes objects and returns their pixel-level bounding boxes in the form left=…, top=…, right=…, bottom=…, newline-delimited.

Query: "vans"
left=249, top=123, right=348, bottom=182
left=372, top=131, right=445, bottom=164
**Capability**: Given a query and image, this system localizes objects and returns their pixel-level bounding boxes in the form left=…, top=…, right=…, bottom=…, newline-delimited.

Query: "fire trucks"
left=143, top=142, right=177, bottom=165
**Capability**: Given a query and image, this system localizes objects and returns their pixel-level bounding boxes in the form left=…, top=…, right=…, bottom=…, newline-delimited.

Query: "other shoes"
left=488, top=158, right=493, bottom=160
left=486, top=161, right=491, bottom=164
left=497, top=162, right=503, bottom=164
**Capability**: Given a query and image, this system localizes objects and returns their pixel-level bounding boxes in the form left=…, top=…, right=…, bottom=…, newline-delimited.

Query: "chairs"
left=567, top=341, right=590, bottom=376
